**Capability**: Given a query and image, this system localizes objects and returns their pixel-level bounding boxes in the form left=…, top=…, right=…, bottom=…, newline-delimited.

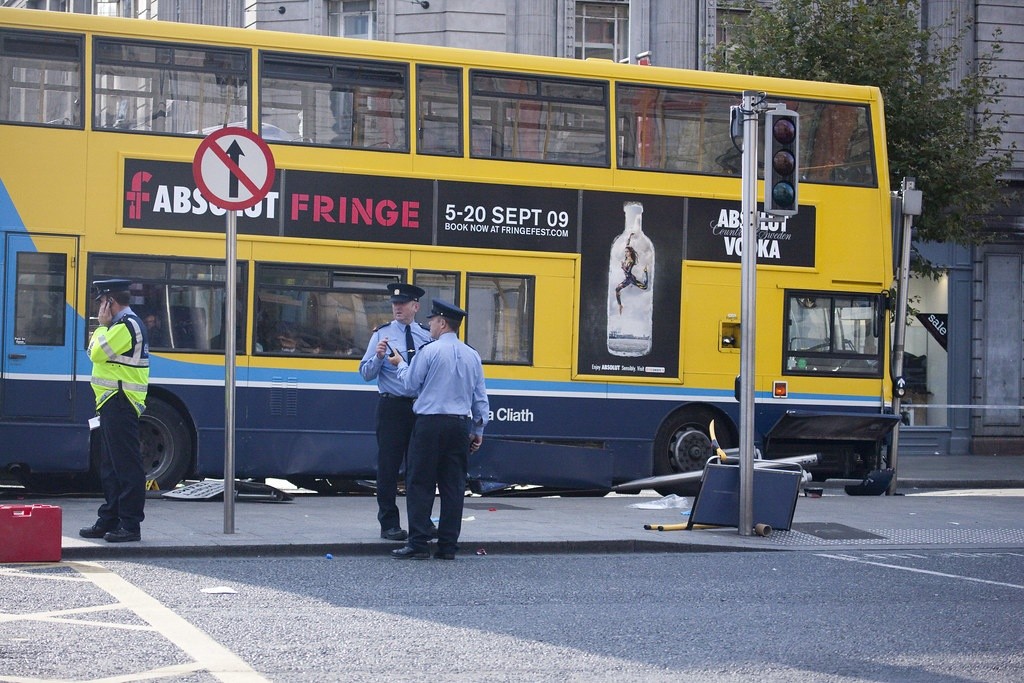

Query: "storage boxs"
left=0, top=504, right=62, bottom=563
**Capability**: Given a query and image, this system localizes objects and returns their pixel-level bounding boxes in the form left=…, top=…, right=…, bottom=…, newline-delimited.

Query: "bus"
left=0, top=5, right=906, bottom=495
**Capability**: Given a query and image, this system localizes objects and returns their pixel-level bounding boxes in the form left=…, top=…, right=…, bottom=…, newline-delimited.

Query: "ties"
left=405, top=324, right=416, bottom=366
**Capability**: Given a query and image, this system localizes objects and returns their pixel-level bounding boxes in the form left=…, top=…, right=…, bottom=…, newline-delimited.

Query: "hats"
left=426, top=299, right=468, bottom=321
left=387, top=283, right=425, bottom=303
left=92, top=279, right=132, bottom=301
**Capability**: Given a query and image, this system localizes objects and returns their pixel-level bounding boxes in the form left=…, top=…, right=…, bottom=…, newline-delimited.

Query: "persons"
left=235, top=312, right=264, bottom=352
left=388, top=300, right=489, bottom=560
left=276, top=321, right=356, bottom=355
left=140, top=311, right=168, bottom=347
left=359, top=283, right=439, bottom=540
left=79, top=279, right=150, bottom=543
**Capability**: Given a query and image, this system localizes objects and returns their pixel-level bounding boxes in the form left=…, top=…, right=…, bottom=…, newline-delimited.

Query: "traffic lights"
left=763, top=107, right=799, bottom=218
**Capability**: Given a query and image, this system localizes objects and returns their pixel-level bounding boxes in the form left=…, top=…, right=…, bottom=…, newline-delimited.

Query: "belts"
left=379, top=393, right=418, bottom=404
left=441, top=414, right=467, bottom=421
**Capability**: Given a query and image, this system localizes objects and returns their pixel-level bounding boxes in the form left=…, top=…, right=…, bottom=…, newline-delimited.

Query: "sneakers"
left=430, top=525, right=439, bottom=537
left=434, top=549, right=455, bottom=558
left=381, top=526, right=407, bottom=540
left=391, top=545, right=431, bottom=559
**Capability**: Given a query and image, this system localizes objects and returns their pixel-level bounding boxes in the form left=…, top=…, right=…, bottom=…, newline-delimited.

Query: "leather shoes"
left=104, top=527, right=141, bottom=541
left=79, top=524, right=119, bottom=538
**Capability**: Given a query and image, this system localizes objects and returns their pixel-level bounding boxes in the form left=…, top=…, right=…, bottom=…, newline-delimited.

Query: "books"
left=88, top=416, right=101, bottom=431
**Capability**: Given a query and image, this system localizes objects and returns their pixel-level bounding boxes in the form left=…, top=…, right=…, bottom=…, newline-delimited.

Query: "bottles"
left=606, top=201, right=655, bottom=357
